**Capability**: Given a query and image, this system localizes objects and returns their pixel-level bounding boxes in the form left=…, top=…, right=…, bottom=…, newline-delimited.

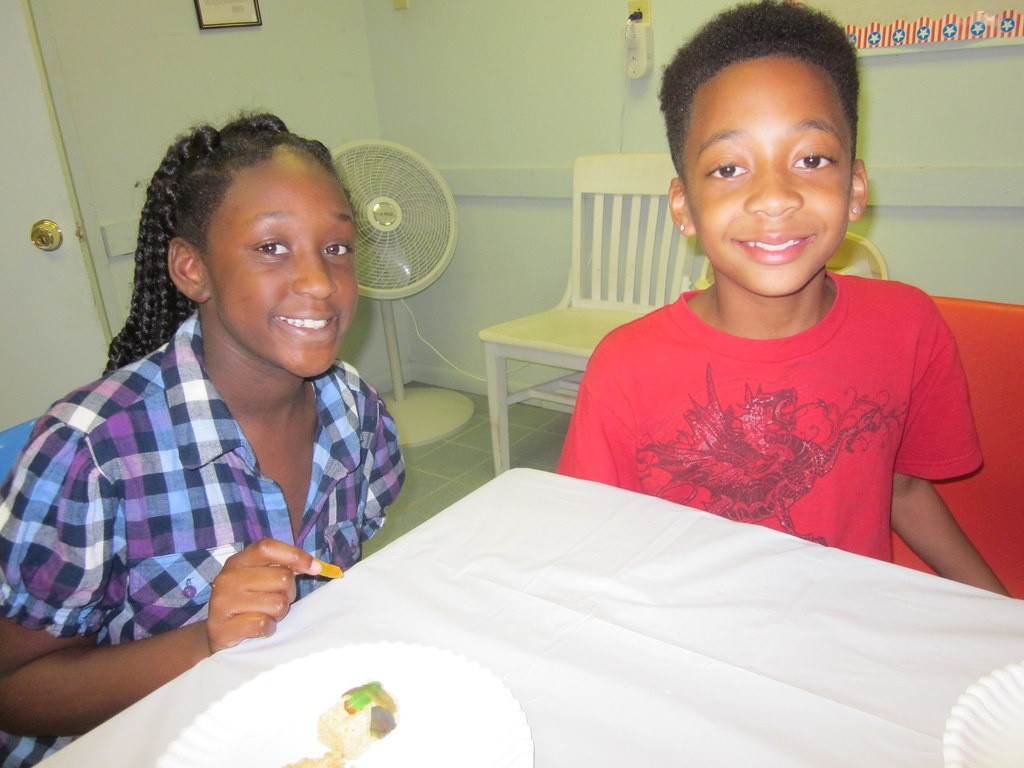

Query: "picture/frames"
left=193, top=0, right=263, bottom=30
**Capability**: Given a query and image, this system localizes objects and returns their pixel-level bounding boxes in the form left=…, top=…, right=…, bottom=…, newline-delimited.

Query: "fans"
left=323, top=137, right=476, bottom=449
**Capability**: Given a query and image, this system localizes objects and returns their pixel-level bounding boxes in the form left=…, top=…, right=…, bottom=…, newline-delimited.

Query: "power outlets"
left=626, top=0, right=651, bottom=25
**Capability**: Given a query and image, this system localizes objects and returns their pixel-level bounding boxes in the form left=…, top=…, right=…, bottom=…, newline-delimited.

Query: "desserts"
left=316, top=680, right=399, bottom=760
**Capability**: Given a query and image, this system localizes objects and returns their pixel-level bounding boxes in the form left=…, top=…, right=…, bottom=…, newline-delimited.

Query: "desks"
left=28, top=466, right=1024, bottom=768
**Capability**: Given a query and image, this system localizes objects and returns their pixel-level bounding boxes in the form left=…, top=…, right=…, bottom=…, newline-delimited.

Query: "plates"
left=156, top=639, right=531, bottom=768
left=937, top=652, right=1024, bottom=768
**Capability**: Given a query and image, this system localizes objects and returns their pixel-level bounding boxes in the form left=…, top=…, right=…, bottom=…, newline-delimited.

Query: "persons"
left=0, top=107, right=406, bottom=768
left=555, top=0, right=1012, bottom=599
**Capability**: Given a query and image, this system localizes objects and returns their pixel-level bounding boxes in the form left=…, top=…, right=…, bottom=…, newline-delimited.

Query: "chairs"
left=476, top=150, right=707, bottom=479
left=887, top=294, right=1024, bottom=603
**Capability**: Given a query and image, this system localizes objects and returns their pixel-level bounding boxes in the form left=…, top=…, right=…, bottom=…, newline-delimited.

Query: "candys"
left=315, top=559, right=344, bottom=578
